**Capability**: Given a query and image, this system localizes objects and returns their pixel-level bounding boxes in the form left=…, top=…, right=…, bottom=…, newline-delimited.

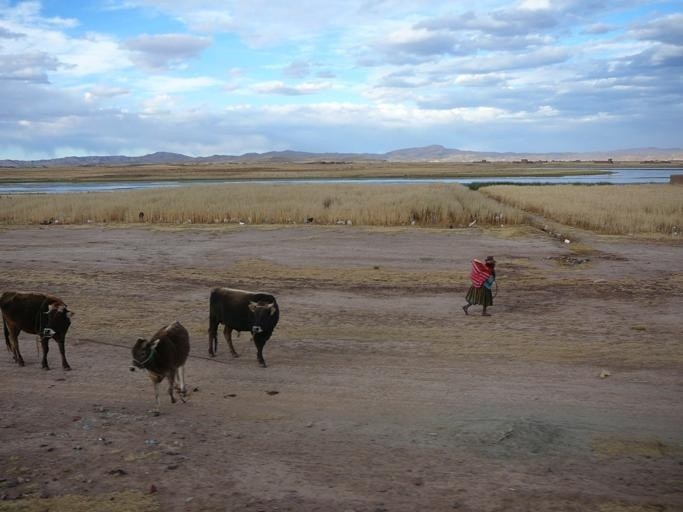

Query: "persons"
left=461, top=255, right=496, bottom=317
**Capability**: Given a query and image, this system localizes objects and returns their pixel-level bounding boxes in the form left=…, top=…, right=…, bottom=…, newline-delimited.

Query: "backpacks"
left=470, top=259, right=490, bottom=287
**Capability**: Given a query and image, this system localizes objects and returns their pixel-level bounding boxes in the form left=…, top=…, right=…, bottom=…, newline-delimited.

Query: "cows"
left=208, top=286, right=280, bottom=369
left=127, top=320, right=191, bottom=417
left=0, top=289, right=75, bottom=372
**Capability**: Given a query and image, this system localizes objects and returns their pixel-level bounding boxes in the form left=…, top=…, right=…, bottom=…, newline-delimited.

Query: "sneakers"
left=463, top=306, right=467, bottom=314
left=482, top=313, right=489, bottom=316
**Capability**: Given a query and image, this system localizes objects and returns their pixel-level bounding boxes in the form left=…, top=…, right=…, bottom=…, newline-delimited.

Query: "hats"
left=485, top=255, right=494, bottom=263
